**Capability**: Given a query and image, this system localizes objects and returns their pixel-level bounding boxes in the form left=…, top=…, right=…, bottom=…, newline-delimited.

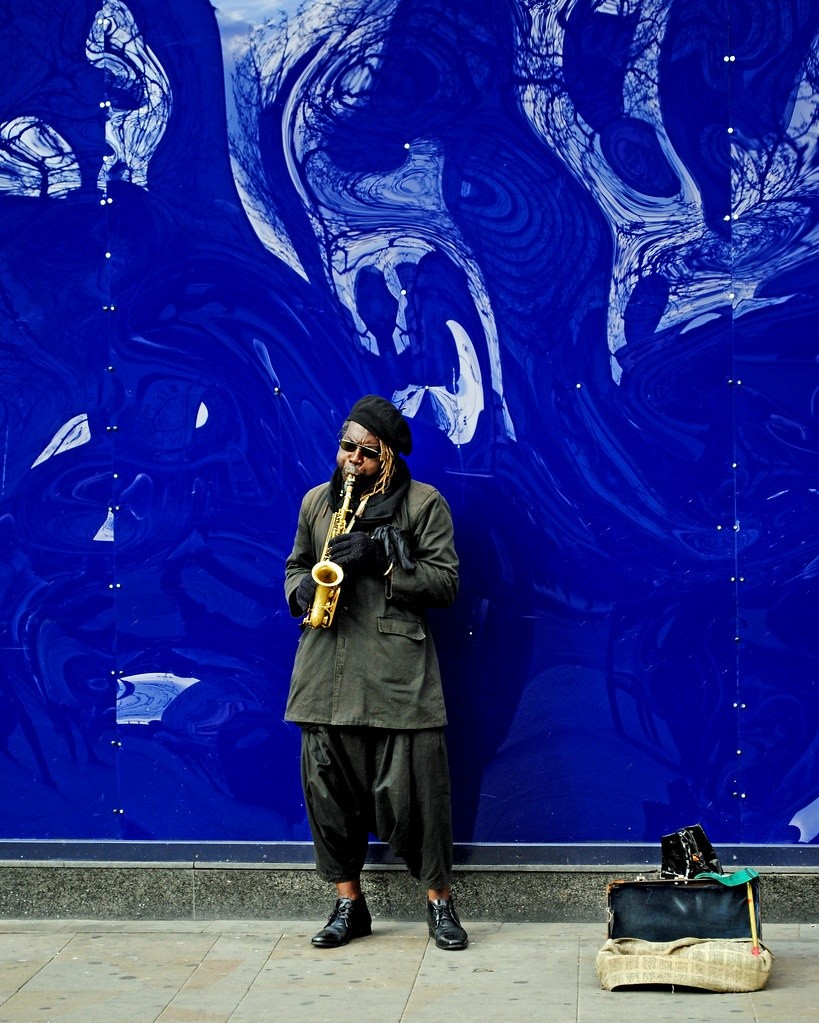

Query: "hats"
left=345, top=395, right=413, bottom=456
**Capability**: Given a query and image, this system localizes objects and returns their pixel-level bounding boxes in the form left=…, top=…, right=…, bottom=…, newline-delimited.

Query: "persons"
left=283, top=396, right=468, bottom=950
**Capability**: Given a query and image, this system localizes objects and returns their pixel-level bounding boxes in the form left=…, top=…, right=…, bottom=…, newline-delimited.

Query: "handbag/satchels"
left=662, top=826, right=724, bottom=880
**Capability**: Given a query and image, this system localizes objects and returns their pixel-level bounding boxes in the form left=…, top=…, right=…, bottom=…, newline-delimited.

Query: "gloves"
left=327, top=532, right=386, bottom=572
left=296, top=574, right=318, bottom=611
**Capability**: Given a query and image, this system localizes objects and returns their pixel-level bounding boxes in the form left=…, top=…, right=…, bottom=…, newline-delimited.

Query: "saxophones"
left=301, top=472, right=356, bottom=630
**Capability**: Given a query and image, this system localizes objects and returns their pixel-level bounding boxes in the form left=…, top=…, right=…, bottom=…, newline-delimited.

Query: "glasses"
left=339, top=435, right=382, bottom=460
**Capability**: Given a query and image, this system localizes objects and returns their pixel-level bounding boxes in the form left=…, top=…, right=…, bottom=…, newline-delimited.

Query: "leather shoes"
left=311, top=896, right=372, bottom=948
left=427, top=896, right=469, bottom=950
